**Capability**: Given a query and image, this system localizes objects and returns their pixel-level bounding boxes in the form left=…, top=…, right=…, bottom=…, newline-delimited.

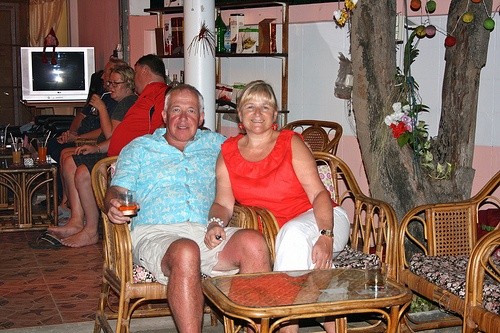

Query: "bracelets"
left=319, top=229, right=334, bottom=237
left=95, top=143, right=101, bottom=154
left=206, top=217, right=225, bottom=228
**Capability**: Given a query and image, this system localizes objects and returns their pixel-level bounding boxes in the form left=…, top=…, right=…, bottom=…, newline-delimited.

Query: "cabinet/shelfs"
left=143, top=2, right=290, bottom=135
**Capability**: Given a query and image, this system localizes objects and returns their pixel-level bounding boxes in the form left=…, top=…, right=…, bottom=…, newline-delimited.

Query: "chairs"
left=93, top=118, right=500, bottom=333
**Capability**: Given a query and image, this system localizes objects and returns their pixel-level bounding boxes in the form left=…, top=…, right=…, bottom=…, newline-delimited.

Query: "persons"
left=103, top=84, right=272, bottom=333
left=204, top=80, right=348, bottom=333
left=47, top=52, right=170, bottom=248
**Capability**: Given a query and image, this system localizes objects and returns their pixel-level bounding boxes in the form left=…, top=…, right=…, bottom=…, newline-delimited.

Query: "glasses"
left=106, top=80, right=128, bottom=87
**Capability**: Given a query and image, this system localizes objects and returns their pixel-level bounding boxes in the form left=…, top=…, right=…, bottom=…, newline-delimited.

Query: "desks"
left=0, top=147, right=58, bottom=232
left=19, top=100, right=87, bottom=116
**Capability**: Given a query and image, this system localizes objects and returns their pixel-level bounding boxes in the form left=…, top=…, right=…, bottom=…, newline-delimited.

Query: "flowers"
left=385, top=29, right=433, bottom=171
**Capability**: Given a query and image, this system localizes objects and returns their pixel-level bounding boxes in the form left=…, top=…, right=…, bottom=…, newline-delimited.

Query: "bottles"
left=215, top=8, right=228, bottom=52
left=166, top=71, right=184, bottom=86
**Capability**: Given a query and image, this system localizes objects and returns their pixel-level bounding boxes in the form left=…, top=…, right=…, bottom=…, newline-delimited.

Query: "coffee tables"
left=201, top=268, right=413, bottom=333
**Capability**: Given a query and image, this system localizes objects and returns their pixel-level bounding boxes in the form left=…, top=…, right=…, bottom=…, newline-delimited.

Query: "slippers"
left=30, top=230, right=65, bottom=249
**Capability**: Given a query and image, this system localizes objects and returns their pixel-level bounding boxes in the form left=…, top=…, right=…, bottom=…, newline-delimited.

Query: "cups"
left=364, top=262, right=387, bottom=294
left=0, top=130, right=5, bottom=146
left=11, top=143, right=21, bottom=163
left=118, top=190, right=137, bottom=216
left=90, top=105, right=98, bottom=116
left=37, top=137, right=48, bottom=165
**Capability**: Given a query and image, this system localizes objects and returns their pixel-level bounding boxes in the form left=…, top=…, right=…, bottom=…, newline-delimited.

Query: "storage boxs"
left=238, top=25, right=259, bottom=53
left=259, top=18, right=277, bottom=53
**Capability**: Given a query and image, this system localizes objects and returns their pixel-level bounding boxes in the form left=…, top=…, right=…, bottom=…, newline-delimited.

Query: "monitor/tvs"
left=20, top=46, right=96, bottom=101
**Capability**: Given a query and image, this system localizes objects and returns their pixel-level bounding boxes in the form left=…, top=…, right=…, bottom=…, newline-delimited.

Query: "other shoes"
left=51, top=206, right=72, bottom=228
